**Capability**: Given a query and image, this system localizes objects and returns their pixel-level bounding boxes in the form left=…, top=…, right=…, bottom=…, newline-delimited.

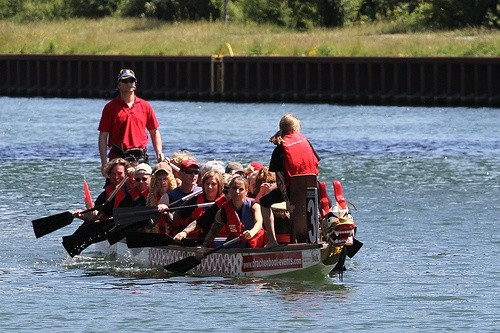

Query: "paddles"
left=32, top=205, right=100, bottom=239
left=114, top=202, right=216, bottom=223
left=56, top=172, right=227, bottom=258
left=162, top=236, right=242, bottom=273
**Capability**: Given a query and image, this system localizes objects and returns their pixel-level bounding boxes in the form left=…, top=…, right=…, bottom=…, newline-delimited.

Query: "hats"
left=180, top=160, right=199, bottom=170
left=135, top=163, right=152, bottom=175
left=152, top=161, right=172, bottom=175
left=118, top=68, right=137, bottom=84
left=244, top=162, right=264, bottom=176
left=225, top=162, right=245, bottom=174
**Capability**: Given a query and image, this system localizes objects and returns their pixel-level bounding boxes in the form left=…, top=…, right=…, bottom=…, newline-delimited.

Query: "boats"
left=95, top=179, right=348, bottom=281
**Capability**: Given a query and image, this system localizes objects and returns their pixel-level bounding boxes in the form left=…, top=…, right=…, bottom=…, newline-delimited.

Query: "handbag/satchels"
left=124, top=148, right=146, bottom=162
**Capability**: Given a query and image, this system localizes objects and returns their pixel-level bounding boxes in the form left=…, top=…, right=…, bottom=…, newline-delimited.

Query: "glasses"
left=182, top=170, right=199, bottom=174
left=135, top=176, right=151, bottom=182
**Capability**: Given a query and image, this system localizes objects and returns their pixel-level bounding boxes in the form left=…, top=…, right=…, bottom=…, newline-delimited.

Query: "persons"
left=97, top=69, right=165, bottom=190
left=269, top=113, right=320, bottom=244
left=72, top=151, right=290, bottom=247
